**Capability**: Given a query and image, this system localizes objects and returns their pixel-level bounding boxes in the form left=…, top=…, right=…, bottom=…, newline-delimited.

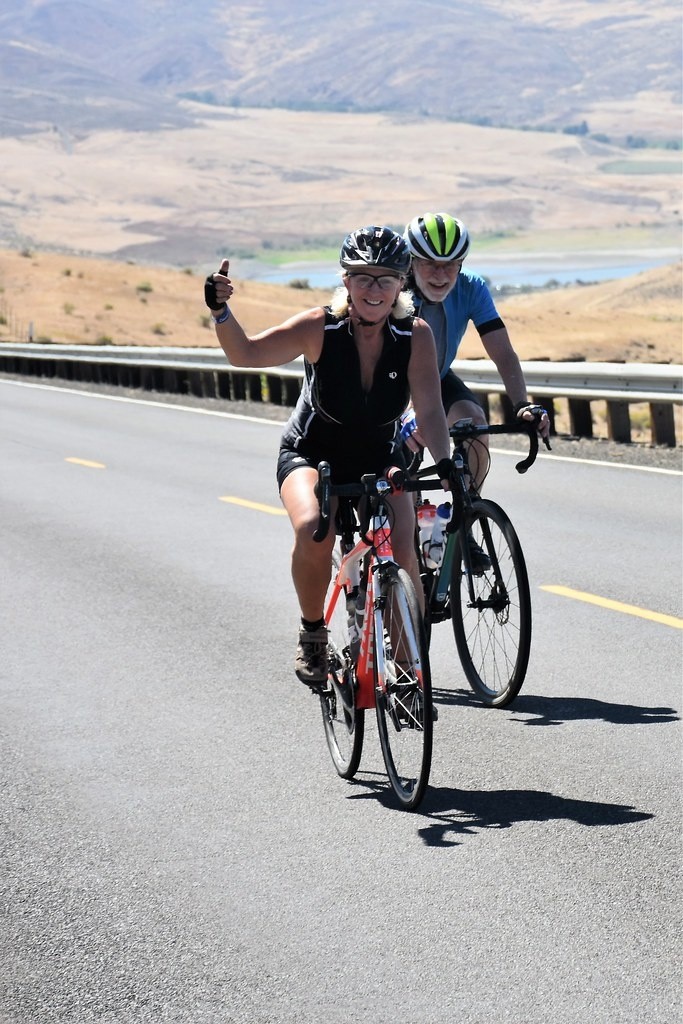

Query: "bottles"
left=427, top=502, right=452, bottom=562
left=369, top=516, right=394, bottom=562
left=417, top=499, right=443, bottom=569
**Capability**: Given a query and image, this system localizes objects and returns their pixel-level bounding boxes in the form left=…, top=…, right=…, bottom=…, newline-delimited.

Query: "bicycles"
left=403, top=412, right=553, bottom=709
left=310, top=456, right=466, bottom=809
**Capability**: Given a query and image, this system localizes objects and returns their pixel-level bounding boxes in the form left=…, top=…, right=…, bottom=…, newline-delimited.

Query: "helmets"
left=403, top=213, right=471, bottom=261
left=340, top=225, right=412, bottom=274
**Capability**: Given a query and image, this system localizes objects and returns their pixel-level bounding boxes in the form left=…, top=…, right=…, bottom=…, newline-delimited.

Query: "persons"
left=203, top=226, right=471, bottom=726
left=390, top=211, right=551, bottom=574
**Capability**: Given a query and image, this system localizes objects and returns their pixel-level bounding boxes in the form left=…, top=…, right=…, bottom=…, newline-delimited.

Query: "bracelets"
left=211, top=306, right=231, bottom=324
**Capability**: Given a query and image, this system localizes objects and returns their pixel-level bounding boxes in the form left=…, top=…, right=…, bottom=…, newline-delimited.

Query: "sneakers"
left=384, top=628, right=393, bottom=655
left=295, top=624, right=331, bottom=686
left=395, top=685, right=438, bottom=721
left=469, top=535, right=490, bottom=570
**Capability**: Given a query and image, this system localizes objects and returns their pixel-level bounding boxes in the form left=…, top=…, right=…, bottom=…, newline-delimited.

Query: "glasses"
left=346, top=272, right=404, bottom=290
left=415, top=258, right=462, bottom=273
